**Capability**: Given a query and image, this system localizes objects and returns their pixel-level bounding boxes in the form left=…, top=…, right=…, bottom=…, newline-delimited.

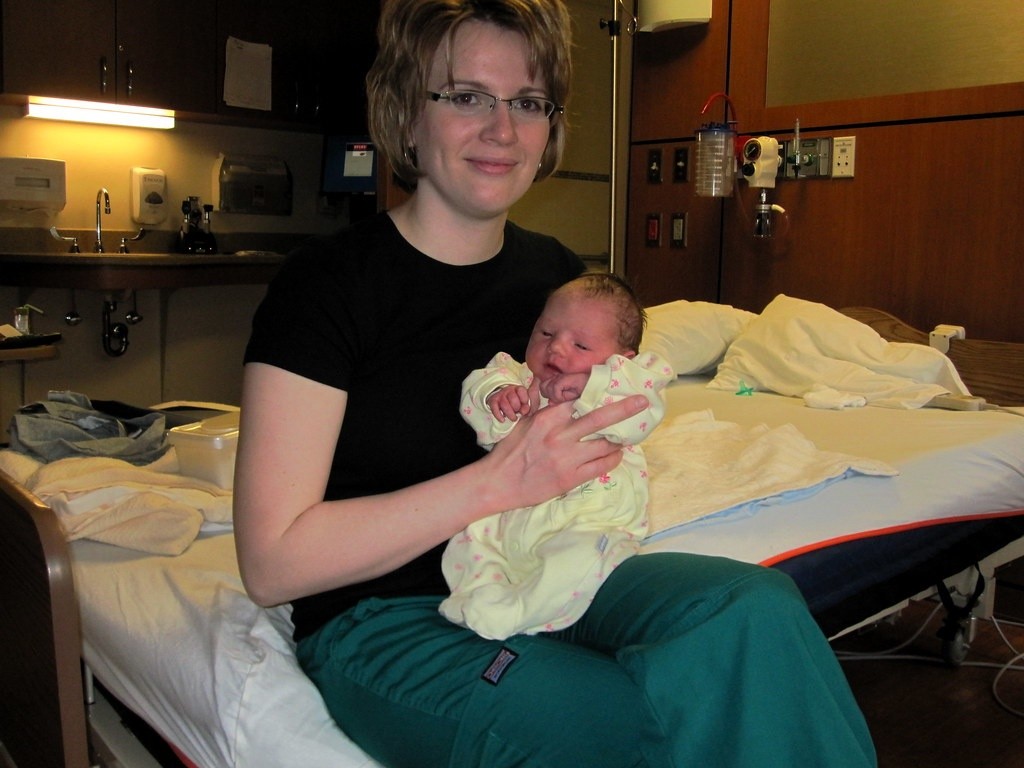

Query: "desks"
left=0, top=345, right=56, bottom=406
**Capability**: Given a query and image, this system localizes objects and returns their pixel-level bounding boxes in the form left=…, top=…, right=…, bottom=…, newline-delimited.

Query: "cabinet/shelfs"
left=0, top=264, right=272, bottom=446
left=0, top=0, right=318, bottom=135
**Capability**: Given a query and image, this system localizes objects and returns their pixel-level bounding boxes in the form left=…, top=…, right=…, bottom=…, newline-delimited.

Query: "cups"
left=15, top=308, right=30, bottom=335
left=692, top=129, right=736, bottom=197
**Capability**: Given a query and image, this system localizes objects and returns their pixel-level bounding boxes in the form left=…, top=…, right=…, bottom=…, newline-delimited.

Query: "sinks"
left=48, top=252, right=172, bottom=258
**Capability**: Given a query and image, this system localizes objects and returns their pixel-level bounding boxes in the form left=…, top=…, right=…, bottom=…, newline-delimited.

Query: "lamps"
left=637, top=0, right=712, bottom=34
left=692, top=121, right=738, bottom=200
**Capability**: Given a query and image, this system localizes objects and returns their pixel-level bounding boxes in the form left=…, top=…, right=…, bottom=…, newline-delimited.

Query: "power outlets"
left=644, top=213, right=663, bottom=247
left=670, top=210, right=688, bottom=248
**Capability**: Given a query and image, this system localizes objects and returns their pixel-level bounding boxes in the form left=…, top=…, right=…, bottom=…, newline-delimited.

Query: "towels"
left=643, top=409, right=901, bottom=537
left=0, top=446, right=233, bottom=556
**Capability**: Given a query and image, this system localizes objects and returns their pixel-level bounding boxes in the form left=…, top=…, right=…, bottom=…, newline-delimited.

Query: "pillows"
left=634, top=301, right=762, bottom=378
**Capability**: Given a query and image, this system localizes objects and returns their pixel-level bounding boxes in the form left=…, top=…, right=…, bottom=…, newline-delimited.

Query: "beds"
left=0, top=306, right=1024, bottom=768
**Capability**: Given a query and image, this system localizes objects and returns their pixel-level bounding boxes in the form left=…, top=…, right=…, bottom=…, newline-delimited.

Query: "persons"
left=437, top=272, right=676, bottom=642
left=231, top=0, right=880, bottom=768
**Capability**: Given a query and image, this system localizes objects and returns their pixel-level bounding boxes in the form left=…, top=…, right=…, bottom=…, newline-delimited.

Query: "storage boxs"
left=167, top=410, right=241, bottom=492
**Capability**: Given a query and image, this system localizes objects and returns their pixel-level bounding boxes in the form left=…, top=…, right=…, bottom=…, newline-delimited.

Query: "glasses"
left=424, top=88, right=565, bottom=124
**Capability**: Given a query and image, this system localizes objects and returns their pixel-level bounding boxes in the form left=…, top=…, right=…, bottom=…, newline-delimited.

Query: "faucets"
left=94, top=187, right=110, bottom=253
